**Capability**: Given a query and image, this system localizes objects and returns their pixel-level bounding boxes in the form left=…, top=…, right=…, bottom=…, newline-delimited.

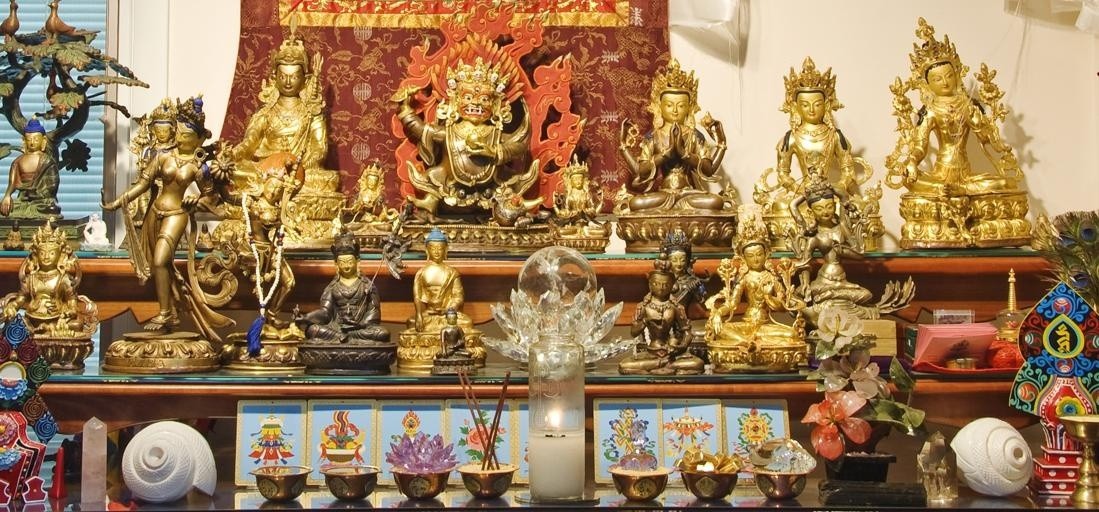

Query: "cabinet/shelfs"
left=0, top=256, right=1054, bottom=509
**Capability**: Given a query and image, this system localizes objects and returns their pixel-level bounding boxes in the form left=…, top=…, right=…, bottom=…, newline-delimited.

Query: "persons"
left=0, top=37, right=523, bottom=383
left=615, top=11, right=1053, bottom=381
left=550, top=156, right=609, bottom=238
